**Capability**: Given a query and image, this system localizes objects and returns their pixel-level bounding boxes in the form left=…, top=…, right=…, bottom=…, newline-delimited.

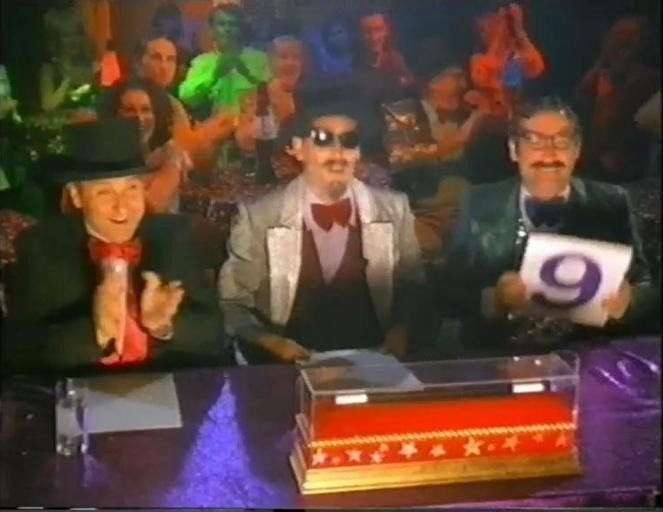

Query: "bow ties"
left=310, top=198, right=350, bottom=230
left=524, top=197, right=572, bottom=229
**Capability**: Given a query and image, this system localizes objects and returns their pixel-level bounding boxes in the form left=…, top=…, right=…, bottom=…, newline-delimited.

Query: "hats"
left=285, top=81, right=379, bottom=140
left=45, top=119, right=160, bottom=181
left=400, top=33, right=462, bottom=84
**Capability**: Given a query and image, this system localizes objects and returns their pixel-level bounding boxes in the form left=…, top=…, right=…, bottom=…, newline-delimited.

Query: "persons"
left=9, top=171, right=219, bottom=376
left=218, top=108, right=423, bottom=373
left=3, top=0, right=663, bottom=236
left=428, top=87, right=655, bottom=357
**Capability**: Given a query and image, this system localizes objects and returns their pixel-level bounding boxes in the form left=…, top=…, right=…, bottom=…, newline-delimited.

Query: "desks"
left=0, top=334, right=662, bottom=509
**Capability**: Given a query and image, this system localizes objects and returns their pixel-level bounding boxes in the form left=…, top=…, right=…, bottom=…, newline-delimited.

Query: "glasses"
left=311, top=128, right=361, bottom=148
left=516, top=131, right=572, bottom=148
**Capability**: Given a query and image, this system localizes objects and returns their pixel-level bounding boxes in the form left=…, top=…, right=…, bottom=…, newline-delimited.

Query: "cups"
left=51, top=375, right=91, bottom=460
